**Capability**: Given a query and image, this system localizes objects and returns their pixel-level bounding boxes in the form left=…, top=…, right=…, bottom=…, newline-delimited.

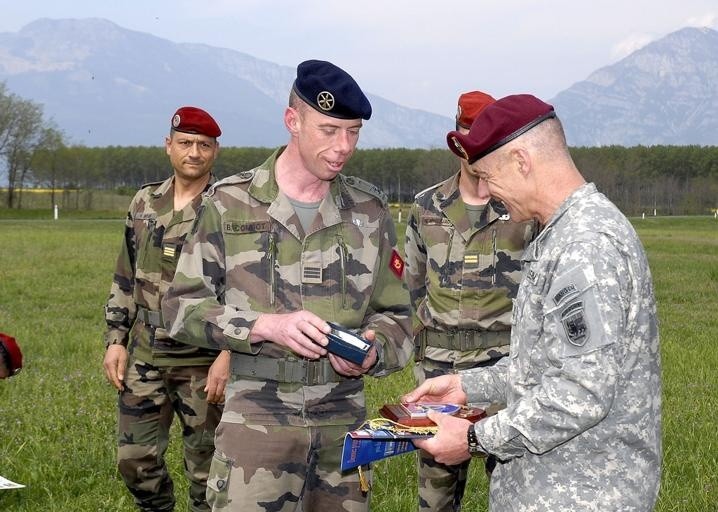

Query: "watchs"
left=468, top=425, right=488, bottom=458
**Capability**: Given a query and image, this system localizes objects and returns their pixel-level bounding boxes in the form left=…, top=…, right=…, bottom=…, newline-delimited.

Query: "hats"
left=171, top=106, right=221, bottom=137
left=293, top=60, right=372, bottom=120
left=446, top=90, right=556, bottom=165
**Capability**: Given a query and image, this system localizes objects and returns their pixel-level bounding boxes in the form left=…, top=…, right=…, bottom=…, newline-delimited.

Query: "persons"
left=400, top=93, right=662, bottom=510
left=104, top=106, right=238, bottom=512
left=405, top=92, right=542, bottom=511
left=160, top=59, right=413, bottom=511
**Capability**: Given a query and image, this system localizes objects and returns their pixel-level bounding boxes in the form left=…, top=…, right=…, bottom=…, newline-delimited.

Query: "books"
left=341, top=431, right=435, bottom=472
